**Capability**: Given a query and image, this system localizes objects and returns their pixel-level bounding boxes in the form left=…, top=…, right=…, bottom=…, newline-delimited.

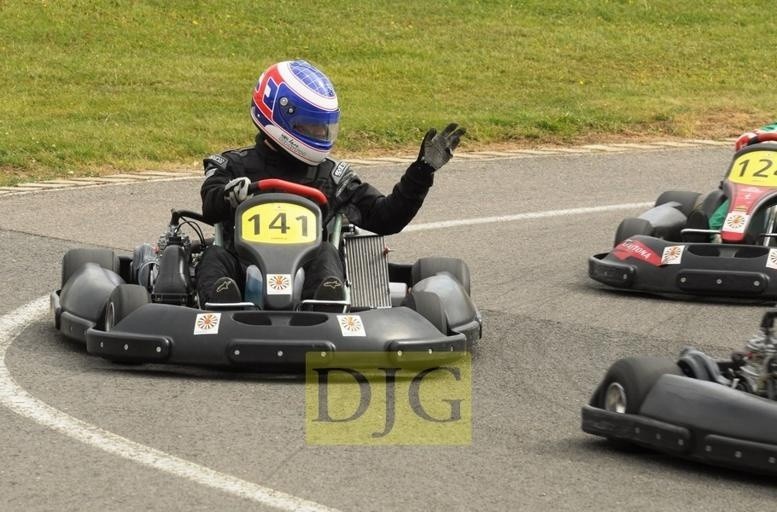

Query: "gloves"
left=221, top=175, right=254, bottom=210
left=733, top=128, right=759, bottom=153
left=413, top=118, right=469, bottom=174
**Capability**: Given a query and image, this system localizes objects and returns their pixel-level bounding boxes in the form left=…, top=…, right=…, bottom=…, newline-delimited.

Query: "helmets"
left=245, top=54, right=344, bottom=172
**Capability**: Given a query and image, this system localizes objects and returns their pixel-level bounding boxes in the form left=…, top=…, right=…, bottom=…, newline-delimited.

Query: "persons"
left=196, top=60, right=465, bottom=312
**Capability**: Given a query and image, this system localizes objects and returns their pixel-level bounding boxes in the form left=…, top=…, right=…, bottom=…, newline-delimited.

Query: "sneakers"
left=682, top=208, right=710, bottom=245
left=207, top=275, right=242, bottom=313
left=309, top=274, right=345, bottom=314
left=674, top=346, right=744, bottom=392
